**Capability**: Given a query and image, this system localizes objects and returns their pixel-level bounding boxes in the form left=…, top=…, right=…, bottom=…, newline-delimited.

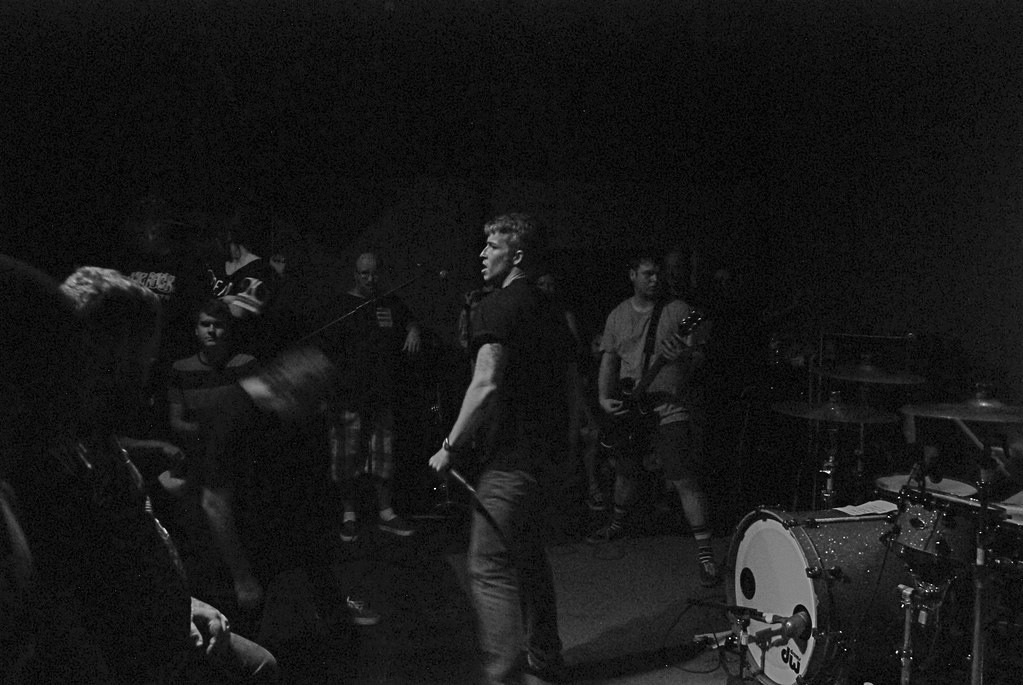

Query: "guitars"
left=592, top=308, right=707, bottom=435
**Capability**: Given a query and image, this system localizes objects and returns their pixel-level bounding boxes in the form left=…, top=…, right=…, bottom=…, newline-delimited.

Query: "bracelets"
left=441, top=437, right=460, bottom=453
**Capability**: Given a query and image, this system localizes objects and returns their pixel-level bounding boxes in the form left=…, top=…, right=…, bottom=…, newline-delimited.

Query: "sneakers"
left=586, top=523, right=626, bottom=545
left=584, top=488, right=607, bottom=510
left=377, top=513, right=415, bottom=536
left=338, top=513, right=359, bottom=542
left=699, top=558, right=720, bottom=586
left=350, top=608, right=381, bottom=626
left=236, top=574, right=266, bottom=624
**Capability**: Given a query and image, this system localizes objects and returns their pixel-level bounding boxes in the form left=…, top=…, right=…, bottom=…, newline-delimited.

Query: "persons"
left=0, top=208, right=742, bottom=685
left=429, top=213, right=576, bottom=685
left=583, top=254, right=721, bottom=587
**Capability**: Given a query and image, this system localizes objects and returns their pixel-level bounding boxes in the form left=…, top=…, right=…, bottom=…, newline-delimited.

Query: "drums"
left=892, top=485, right=1006, bottom=564
left=726, top=505, right=976, bottom=685
left=983, top=502, right=1023, bottom=569
left=872, top=473, right=979, bottom=507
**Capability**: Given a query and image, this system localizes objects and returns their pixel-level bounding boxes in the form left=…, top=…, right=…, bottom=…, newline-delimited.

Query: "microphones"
left=416, top=263, right=448, bottom=278
left=762, top=610, right=810, bottom=637
left=925, top=435, right=945, bottom=484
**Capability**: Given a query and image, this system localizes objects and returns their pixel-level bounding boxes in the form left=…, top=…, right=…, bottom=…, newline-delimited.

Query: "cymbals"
left=898, top=398, right=1023, bottom=422
left=771, top=399, right=901, bottom=424
left=807, top=359, right=927, bottom=385
